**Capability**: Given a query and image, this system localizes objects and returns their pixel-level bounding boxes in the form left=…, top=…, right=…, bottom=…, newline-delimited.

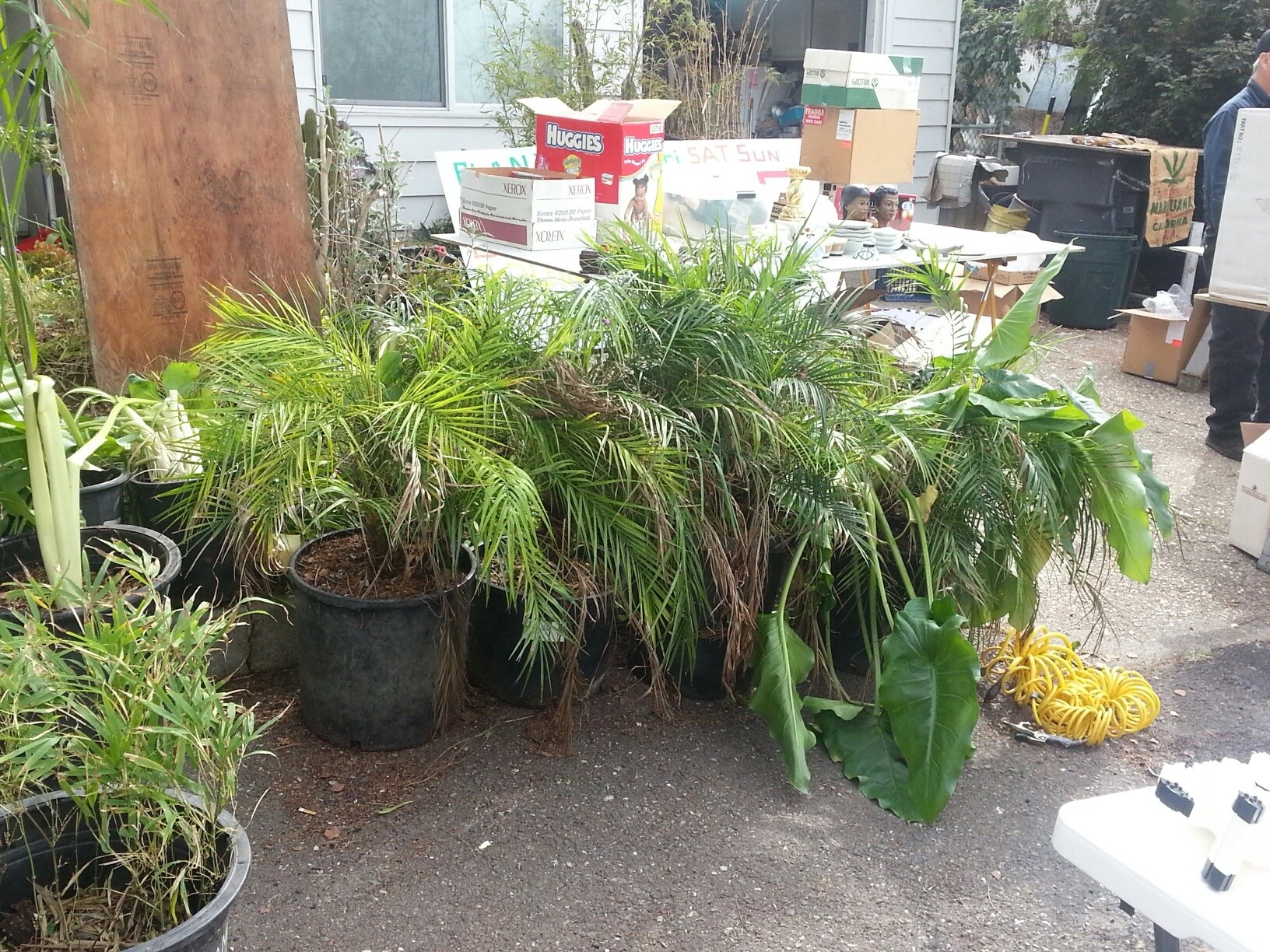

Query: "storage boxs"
left=455, top=167, right=597, bottom=251
left=514, top=98, right=682, bottom=252
left=800, top=47, right=925, bottom=109
left=798, top=103, right=921, bottom=183
left=1227, top=423, right=1270, bottom=576
left=945, top=264, right=1066, bottom=326
left=1107, top=287, right=1216, bottom=385
left=835, top=187, right=916, bottom=249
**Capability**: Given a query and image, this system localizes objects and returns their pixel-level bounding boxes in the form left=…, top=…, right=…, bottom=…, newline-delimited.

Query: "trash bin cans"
left=1050, top=230, right=1138, bottom=329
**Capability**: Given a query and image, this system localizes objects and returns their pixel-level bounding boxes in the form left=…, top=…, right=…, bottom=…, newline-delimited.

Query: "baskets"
left=875, top=263, right=950, bottom=303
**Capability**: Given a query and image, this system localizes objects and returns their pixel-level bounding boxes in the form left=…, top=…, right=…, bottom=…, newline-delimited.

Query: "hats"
left=1256, top=29, right=1270, bottom=59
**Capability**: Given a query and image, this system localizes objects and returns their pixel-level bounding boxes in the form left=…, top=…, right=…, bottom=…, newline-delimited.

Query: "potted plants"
left=0, top=219, right=1176, bottom=952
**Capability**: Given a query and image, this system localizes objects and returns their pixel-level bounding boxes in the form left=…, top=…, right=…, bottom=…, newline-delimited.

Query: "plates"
left=902, top=239, right=965, bottom=258
left=874, top=226, right=904, bottom=254
left=823, top=221, right=874, bottom=241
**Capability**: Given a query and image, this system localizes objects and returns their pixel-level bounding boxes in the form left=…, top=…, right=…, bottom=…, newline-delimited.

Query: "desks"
left=978, top=133, right=1205, bottom=156
left=1052, top=753, right=1269, bottom=951
left=429, top=223, right=1088, bottom=282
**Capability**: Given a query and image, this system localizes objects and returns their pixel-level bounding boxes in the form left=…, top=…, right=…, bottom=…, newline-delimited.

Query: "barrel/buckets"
left=1046, top=229, right=1138, bottom=330
left=984, top=204, right=1030, bottom=234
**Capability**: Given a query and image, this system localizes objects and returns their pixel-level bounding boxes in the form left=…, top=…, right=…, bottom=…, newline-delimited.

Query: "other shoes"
left=1205, top=428, right=1245, bottom=461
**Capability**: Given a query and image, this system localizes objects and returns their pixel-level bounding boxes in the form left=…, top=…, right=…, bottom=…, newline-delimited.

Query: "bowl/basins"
left=844, top=241, right=861, bottom=256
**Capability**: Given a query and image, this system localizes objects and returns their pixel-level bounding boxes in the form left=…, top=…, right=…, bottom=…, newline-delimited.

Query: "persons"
left=1203, top=29, right=1270, bottom=459
left=624, top=174, right=653, bottom=224
left=872, top=184, right=900, bottom=227
left=841, top=183, right=870, bottom=221
